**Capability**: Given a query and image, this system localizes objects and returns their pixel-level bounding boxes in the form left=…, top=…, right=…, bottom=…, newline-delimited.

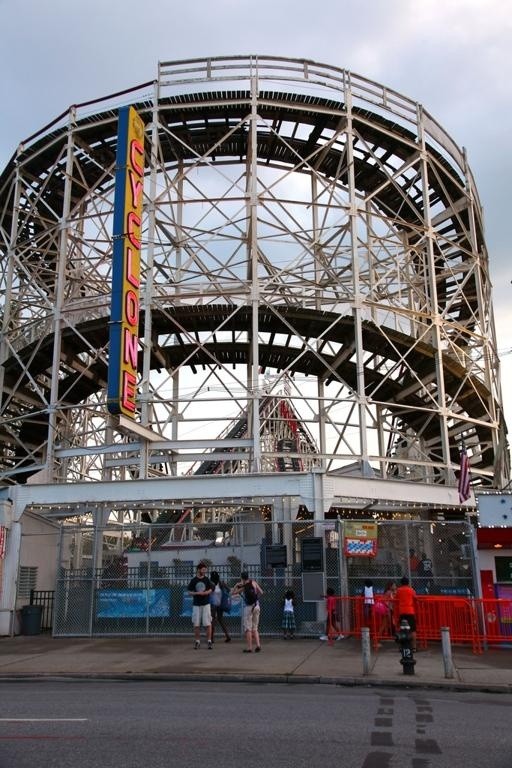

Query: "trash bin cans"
left=21, top=604, right=43, bottom=636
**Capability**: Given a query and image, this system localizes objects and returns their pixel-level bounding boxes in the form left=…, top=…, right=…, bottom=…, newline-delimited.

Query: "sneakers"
left=337, top=636, right=345, bottom=640
left=194, top=638, right=261, bottom=652
left=320, top=635, right=329, bottom=640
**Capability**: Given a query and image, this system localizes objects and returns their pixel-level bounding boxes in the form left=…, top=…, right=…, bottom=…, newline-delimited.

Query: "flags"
left=458, top=432, right=471, bottom=505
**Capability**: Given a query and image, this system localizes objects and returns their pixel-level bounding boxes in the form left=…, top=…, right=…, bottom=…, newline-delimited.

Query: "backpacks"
left=220, top=582, right=231, bottom=612
left=238, top=581, right=257, bottom=605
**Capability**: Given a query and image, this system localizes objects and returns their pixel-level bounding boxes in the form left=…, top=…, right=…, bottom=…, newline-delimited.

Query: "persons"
left=209, top=571, right=232, bottom=643
left=188, top=563, right=215, bottom=649
left=364, top=549, right=434, bottom=652
left=280, top=590, right=297, bottom=640
left=232, top=571, right=263, bottom=653
left=319, top=588, right=345, bottom=641
